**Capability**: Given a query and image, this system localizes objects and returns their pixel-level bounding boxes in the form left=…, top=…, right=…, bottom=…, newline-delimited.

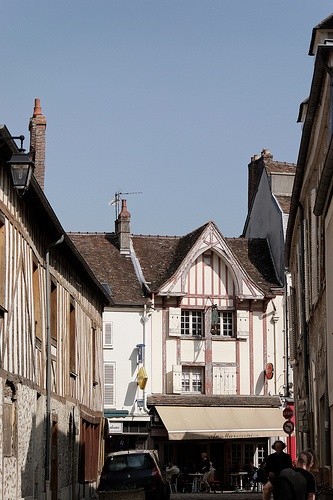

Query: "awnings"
left=154, top=405, right=296, bottom=440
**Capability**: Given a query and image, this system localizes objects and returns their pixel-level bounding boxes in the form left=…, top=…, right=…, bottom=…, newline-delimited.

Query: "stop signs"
left=283, top=409, right=293, bottom=419
left=265, top=363, right=273, bottom=379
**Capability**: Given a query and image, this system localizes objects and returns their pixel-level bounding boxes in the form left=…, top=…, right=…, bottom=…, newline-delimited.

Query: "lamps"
left=0, top=134, right=36, bottom=196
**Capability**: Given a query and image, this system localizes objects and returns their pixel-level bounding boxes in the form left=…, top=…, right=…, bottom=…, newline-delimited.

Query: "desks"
left=189, top=474, right=204, bottom=493
left=230, top=474, right=244, bottom=491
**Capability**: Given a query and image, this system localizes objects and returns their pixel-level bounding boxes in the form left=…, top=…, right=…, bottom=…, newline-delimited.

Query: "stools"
left=209, top=481, right=223, bottom=494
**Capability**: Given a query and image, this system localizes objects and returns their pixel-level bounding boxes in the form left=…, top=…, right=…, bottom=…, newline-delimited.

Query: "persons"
left=166, top=464, right=180, bottom=492
left=200, top=462, right=215, bottom=492
left=242, top=460, right=258, bottom=491
left=263, top=451, right=318, bottom=500
left=255, top=462, right=269, bottom=486
left=202, top=452, right=211, bottom=493
left=266, top=440, right=292, bottom=482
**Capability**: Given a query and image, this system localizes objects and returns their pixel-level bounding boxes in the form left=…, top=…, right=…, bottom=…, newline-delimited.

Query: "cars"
left=98, top=450, right=171, bottom=500
